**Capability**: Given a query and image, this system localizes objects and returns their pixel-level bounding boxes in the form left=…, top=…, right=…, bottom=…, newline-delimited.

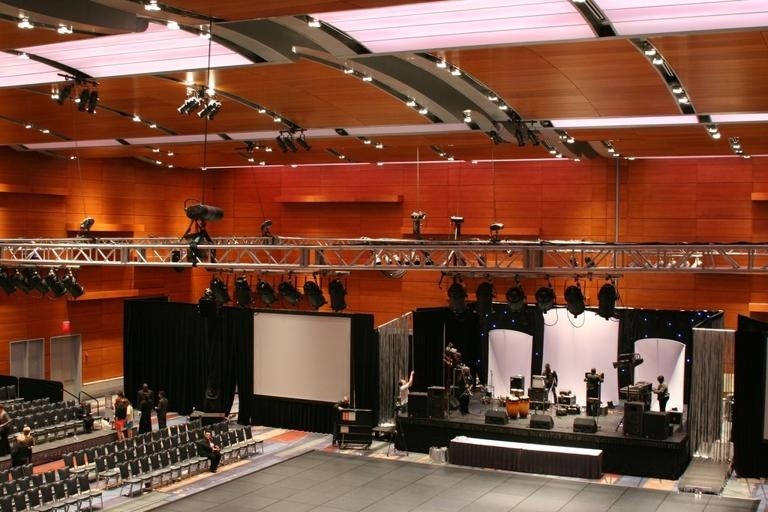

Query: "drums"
left=518, top=396, right=530, bottom=418
left=507, top=397, right=519, bottom=419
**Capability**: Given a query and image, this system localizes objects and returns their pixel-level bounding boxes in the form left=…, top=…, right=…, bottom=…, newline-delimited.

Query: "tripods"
left=386, top=412, right=408, bottom=457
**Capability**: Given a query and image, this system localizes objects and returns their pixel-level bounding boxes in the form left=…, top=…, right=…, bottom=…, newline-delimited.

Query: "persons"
left=542, top=362, right=559, bottom=406
left=456, top=366, right=475, bottom=416
left=651, top=373, right=671, bottom=413
left=332, top=396, right=352, bottom=448
left=0, top=402, right=12, bottom=458
left=77, top=398, right=94, bottom=434
left=11, top=425, right=35, bottom=469
left=198, top=431, right=222, bottom=473
left=111, top=382, right=168, bottom=446
left=398, top=369, right=416, bottom=413
left=584, top=368, right=605, bottom=417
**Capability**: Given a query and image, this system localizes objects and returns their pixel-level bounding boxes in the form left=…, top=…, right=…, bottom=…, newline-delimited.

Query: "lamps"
left=272, top=125, right=316, bottom=154
left=175, top=93, right=222, bottom=122
left=186, top=270, right=355, bottom=316
left=1, top=264, right=91, bottom=307
left=175, top=193, right=232, bottom=261
left=75, top=215, right=96, bottom=239
left=484, top=116, right=542, bottom=148
left=403, top=206, right=509, bottom=246
left=442, top=275, right=624, bottom=321
left=256, top=216, right=279, bottom=244
left=47, top=71, right=104, bottom=116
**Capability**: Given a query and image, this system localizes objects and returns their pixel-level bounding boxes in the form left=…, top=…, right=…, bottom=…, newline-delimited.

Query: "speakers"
left=485, top=409, right=509, bottom=425
left=573, top=417, right=597, bottom=433
left=428, top=386, right=445, bottom=417
left=624, top=401, right=644, bottom=437
left=408, top=392, right=428, bottom=417
left=644, top=411, right=669, bottom=440
left=530, top=413, right=554, bottom=430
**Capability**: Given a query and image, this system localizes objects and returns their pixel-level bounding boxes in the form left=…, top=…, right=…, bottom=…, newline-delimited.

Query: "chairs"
left=0, top=385, right=264, bottom=512
